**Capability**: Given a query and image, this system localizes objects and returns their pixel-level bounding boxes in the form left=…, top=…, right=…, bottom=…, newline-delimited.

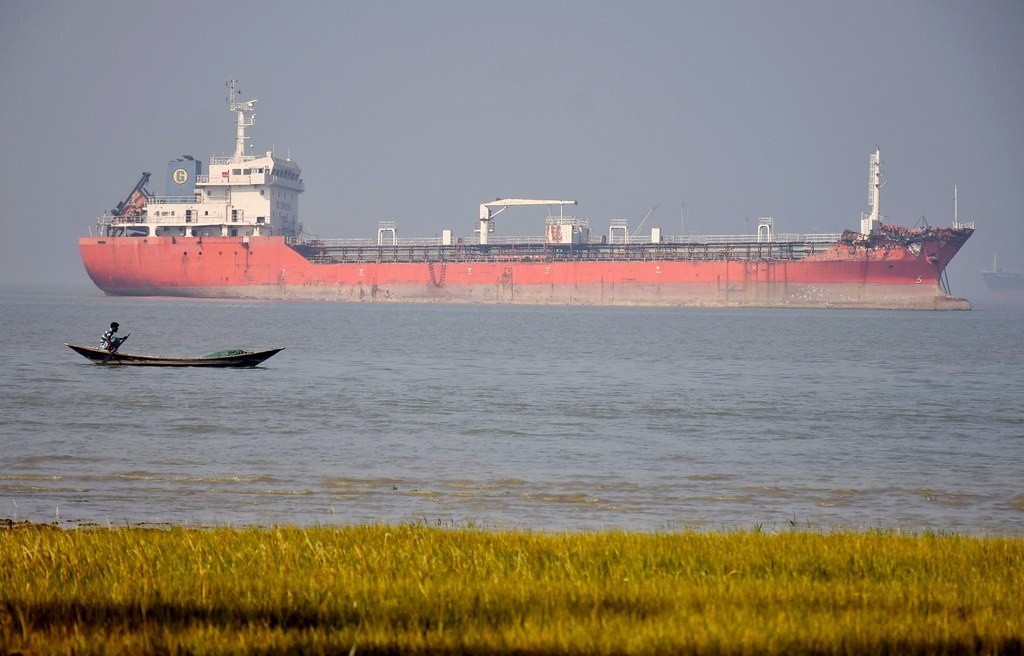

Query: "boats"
left=76, top=76, right=977, bottom=312
left=980, top=254, right=1023, bottom=291
left=63, top=340, right=285, bottom=369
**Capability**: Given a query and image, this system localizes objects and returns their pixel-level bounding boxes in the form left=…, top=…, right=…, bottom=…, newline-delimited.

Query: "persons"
left=101, top=321, right=121, bottom=354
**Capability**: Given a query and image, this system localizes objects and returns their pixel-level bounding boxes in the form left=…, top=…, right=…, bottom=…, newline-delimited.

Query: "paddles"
left=99, top=331, right=132, bottom=367
